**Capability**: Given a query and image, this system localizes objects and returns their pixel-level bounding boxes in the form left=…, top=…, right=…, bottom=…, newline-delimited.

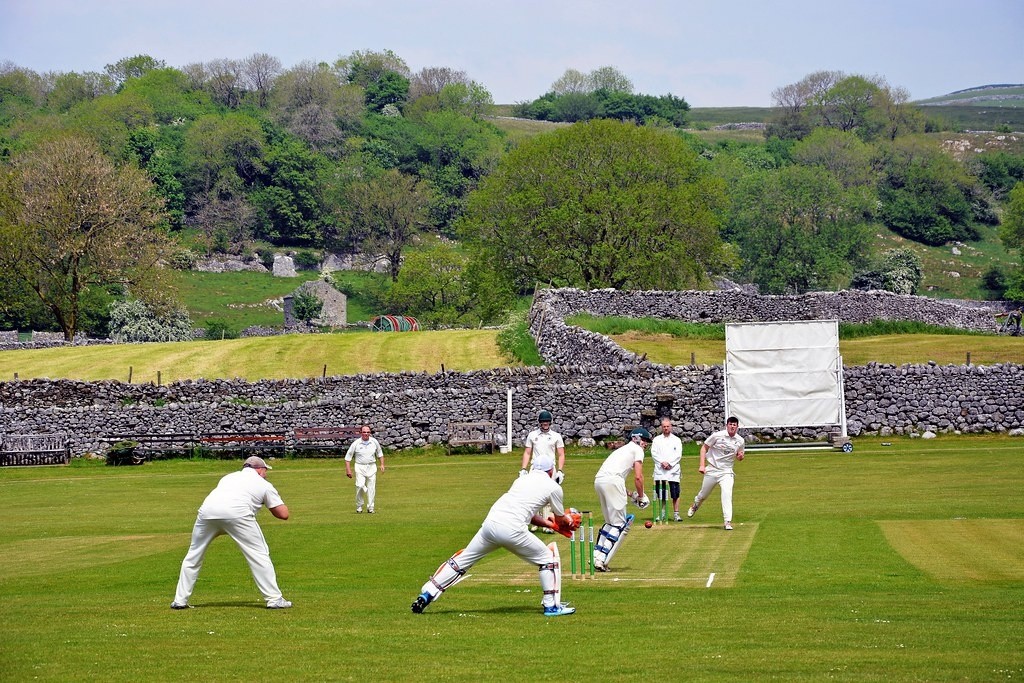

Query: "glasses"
left=362, top=431, right=371, bottom=434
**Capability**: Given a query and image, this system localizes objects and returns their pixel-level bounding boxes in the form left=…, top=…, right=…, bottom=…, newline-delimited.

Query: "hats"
left=538, top=411, right=552, bottom=422
left=629, top=428, right=653, bottom=443
left=534, top=455, right=553, bottom=471
left=243, top=456, right=272, bottom=469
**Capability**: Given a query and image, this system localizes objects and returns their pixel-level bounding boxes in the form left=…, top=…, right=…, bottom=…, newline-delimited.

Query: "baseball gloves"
left=564, top=507, right=582, bottom=531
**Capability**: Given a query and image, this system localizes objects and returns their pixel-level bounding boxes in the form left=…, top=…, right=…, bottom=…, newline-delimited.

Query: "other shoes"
left=368, top=510, right=375, bottom=513
left=656, top=514, right=665, bottom=521
left=267, top=598, right=291, bottom=609
left=724, top=522, right=733, bottom=530
left=687, top=506, right=695, bottom=518
left=674, top=515, right=683, bottom=521
left=357, top=509, right=362, bottom=512
left=170, top=602, right=194, bottom=608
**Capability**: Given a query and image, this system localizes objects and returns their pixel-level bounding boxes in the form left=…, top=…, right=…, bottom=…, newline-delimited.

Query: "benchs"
left=291, top=428, right=361, bottom=460
left=102, top=434, right=195, bottom=461
left=448, top=422, right=497, bottom=456
left=0, top=433, right=72, bottom=467
left=200, top=431, right=287, bottom=460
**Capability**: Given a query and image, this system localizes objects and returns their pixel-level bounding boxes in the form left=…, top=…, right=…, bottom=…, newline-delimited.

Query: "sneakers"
left=588, top=558, right=610, bottom=572
left=410, top=592, right=431, bottom=614
left=544, top=601, right=575, bottom=616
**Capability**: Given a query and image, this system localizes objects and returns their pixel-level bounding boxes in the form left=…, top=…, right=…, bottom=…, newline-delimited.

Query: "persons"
left=518, top=411, right=565, bottom=533
left=171, top=456, right=295, bottom=608
left=411, top=455, right=581, bottom=616
left=344, top=426, right=386, bottom=514
left=588, top=426, right=652, bottom=570
left=685, top=417, right=745, bottom=530
left=650, top=417, right=684, bottom=521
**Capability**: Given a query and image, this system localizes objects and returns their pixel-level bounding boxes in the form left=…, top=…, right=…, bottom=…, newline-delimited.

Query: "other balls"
left=645, top=521, right=652, bottom=528
